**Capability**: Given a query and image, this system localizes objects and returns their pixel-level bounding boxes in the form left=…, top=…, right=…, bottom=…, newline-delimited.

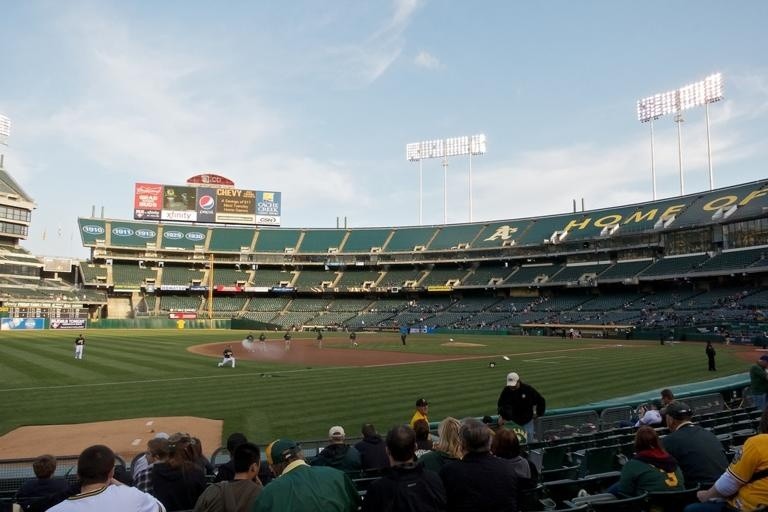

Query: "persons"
left=658, top=388, right=676, bottom=414
left=217, top=342, right=236, bottom=368
left=497, top=371, right=545, bottom=446
left=748, top=353, right=768, bottom=408
left=73, top=333, right=85, bottom=360
left=125, top=415, right=544, bottom=512
left=409, top=398, right=430, bottom=430
left=607, top=388, right=768, bottom=512
left=45, top=443, right=168, bottom=511
left=10, top=453, right=70, bottom=511
left=259, top=332, right=266, bottom=351
left=280, top=289, right=768, bottom=350
left=246, top=331, right=254, bottom=353
left=705, top=340, right=716, bottom=371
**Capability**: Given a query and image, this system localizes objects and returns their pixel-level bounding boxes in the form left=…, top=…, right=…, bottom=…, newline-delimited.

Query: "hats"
left=504, top=372, right=519, bottom=387
left=329, top=426, right=345, bottom=437
left=667, top=401, right=694, bottom=419
left=267, top=438, right=300, bottom=466
left=416, top=399, right=428, bottom=406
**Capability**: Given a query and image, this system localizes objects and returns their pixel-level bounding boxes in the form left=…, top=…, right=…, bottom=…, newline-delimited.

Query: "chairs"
left=390, top=381, right=766, bottom=508
left=367, top=251, right=767, bottom=350
left=4, top=422, right=388, bottom=510
left=2, top=251, right=364, bottom=347
